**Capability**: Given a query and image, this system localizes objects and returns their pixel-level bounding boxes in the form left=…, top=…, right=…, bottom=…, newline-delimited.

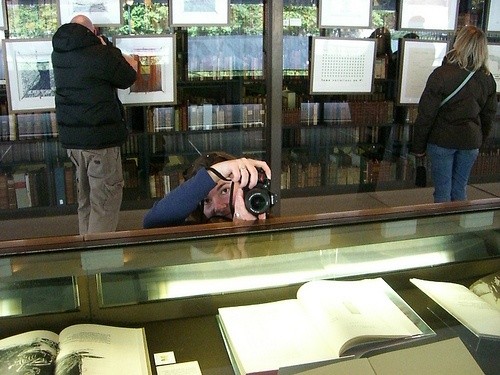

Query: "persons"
left=411, top=23, right=498, bottom=202
left=142, top=152, right=273, bottom=261
left=51, top=15, right=138, bottom=234
left=349, top=26, right=415, bottom=194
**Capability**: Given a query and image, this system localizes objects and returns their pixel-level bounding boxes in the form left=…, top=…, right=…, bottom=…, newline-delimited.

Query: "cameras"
left=243, top=179, right=278, bottom=217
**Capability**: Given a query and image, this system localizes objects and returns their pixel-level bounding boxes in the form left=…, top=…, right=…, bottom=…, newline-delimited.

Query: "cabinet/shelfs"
left=0, top=0, right=500, bottom=221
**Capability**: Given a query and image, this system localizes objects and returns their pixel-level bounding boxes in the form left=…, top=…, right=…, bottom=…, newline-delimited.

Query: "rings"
left=240, top=168, right=246, bottom=172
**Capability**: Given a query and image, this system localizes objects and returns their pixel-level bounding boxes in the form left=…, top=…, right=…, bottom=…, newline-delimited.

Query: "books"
left=294, top=128, right=379, bottom=145
left=282, top=99, right=395, bottom=124
left=120, top=130, right=264, bottom=153
left=282, top=52, right=311, bottom=81
left=392, top=108, right=418, bottom=143
left=125, top=91, right=266, bottom=133
left=280, top=159, right=361, bottom=190
left=120, top=160, right=187, bottom=200
left=470, top=126, right=500, bottom=178
left=360, top=154, right=431, bottom=184
left=0, top=99, right=68, bottom=163
left=343, top=85, right=387, bottom=102
left=0, top=167, right=77, bottom=211
left=187, top=55, right=265, bottom=81
left=0, top=270, right=500, bottom=375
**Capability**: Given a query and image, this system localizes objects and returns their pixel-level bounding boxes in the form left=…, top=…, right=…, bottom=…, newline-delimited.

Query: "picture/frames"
left=112, top=34, right=177, bottom=106
left=57, top=0, right=123, bottom=29
left=168, top=0, right=230, bottom=27
left=2, top=39, right=57, bottom=113
